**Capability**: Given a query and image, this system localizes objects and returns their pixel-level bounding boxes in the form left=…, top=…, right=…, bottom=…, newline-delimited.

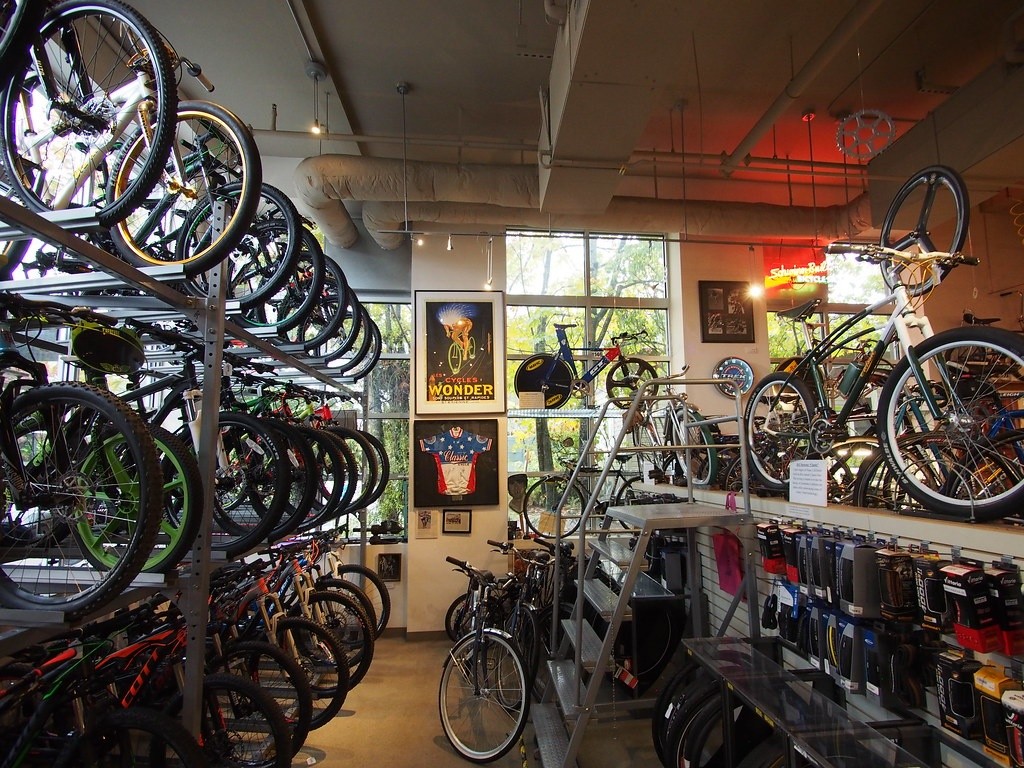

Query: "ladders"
left=526, top=379, right=762, bottom=767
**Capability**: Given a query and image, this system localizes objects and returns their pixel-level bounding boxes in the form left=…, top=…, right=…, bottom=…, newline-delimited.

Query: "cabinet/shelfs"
left=0, top=194, right=370, bottom=768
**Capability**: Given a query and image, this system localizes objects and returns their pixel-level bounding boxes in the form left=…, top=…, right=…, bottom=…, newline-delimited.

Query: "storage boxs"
left=755, top=522, right=1024, bottom=768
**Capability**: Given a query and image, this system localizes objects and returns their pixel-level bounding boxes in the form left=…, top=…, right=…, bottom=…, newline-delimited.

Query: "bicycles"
left=513, top=324, right=659, bottom=414
left=432, top=167, right=1024, bottom=764
left=1, top=0, right=391, bottom=768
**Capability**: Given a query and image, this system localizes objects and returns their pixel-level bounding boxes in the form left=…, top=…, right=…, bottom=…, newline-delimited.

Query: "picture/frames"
left=414, top=288, right=506, bottom=416
left=378, top=552, right=403, bottom=582
left=443, top=509, right=472, bottom=534
left=697, top=280, right=756, bottom=344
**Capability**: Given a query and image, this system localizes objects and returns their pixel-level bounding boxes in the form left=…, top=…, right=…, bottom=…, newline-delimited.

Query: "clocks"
left=712, top=356, right=754, bottom=398
